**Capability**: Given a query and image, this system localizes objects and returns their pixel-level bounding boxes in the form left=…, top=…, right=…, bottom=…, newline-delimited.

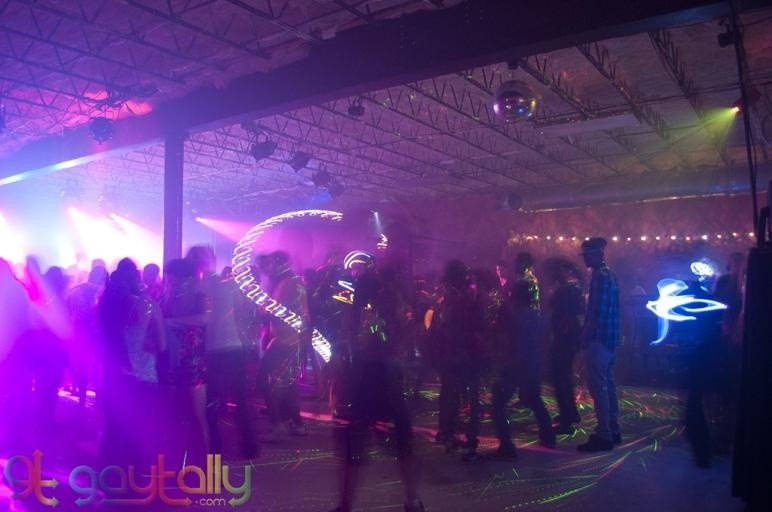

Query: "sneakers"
left=437, top=414, right=621, bottom=465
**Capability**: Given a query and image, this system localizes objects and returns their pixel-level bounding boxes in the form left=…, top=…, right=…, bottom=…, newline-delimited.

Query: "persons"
left=0, top=235, right=748, bottom=508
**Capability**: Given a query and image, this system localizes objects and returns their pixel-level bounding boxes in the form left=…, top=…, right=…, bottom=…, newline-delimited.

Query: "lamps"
left=90, top=91, right=362, bottom=199
left=494, top=63, right=536, bottom=122
left=494, top=186, right=521, bottom=213
